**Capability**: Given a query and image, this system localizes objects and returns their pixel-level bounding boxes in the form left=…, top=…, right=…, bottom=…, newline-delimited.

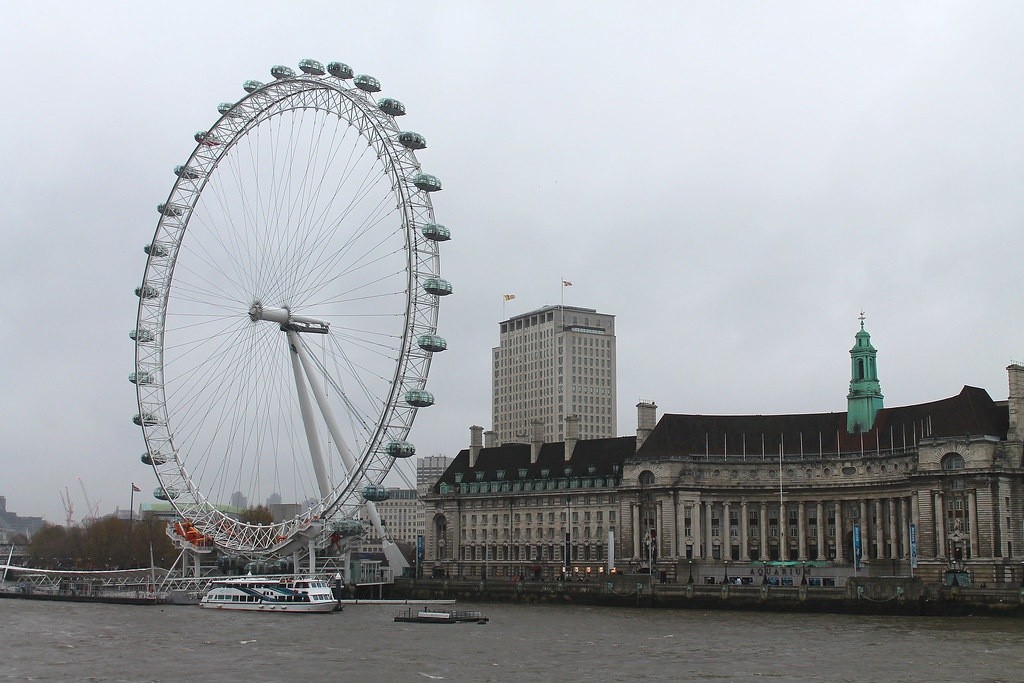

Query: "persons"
left=706, top=577, right=749, bottom=585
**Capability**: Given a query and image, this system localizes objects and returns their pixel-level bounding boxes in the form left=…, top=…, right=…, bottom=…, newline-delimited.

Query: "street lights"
left=480, top=560, right=486, bottom=580
left=519, top=560, right=525, bottom=581
left=561, top=560, right=566, bottom=581
left=688, top=561, right=694, bottom=583
left=412, top=560, right=416, bottom=579
left=799, top=560, right=809, bottom=585
left=950, top=558, right=960, bottom=587
left=722, top=560, right=730, bottom=584
left=445, top=560, right=450, bottom=579
left=761, top=560, right=769, bottom=584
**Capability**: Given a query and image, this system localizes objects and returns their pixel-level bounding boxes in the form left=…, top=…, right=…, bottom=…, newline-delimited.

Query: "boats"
left=199, top=574, right=339, bottom=614
left=165, top=590, right=201, bottom=606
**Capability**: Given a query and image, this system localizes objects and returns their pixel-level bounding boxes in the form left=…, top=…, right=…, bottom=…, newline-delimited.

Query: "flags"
left=563, top=280, right=572, bottom=286
left=503, top=295, right=515, bottom=301
